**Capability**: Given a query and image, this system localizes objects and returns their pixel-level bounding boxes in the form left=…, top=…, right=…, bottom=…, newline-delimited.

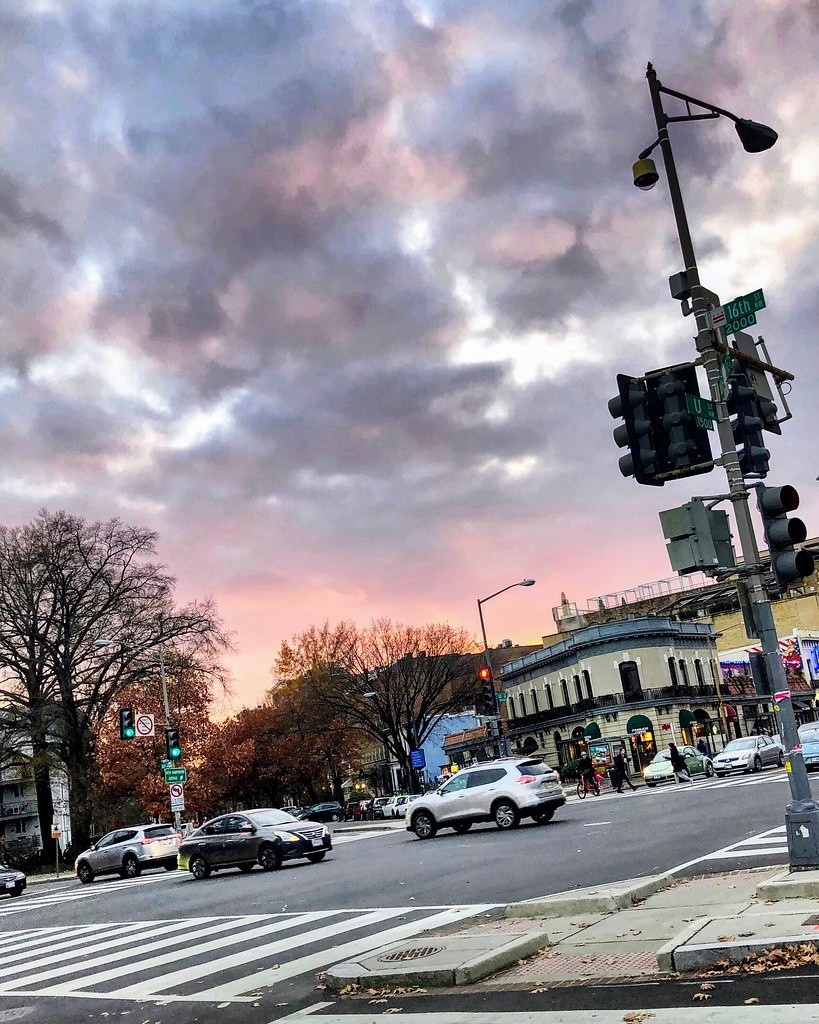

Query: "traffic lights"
left=608, top=374, right=668, bottom=486
left=167, top=728, right=182, bottom=760
left=751, top=481, right=817, bottom=592
left=477, top=665, right=497, bottom=714
left=120, top=707, right=135, bottom=741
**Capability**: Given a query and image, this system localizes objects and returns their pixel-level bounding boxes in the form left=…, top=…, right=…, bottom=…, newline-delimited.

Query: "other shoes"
left=634, top=785, right=638, bottom=791
left=690, top=778, right=693, bottom=786
left=617, top=790, right=624, bottom=793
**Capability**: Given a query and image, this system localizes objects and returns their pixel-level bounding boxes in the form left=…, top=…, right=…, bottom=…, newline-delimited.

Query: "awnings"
left=627, top=715, right=654, bottom=733
left=583, top=722, right=601, bottom=741
left=723, top=703, right=737, bottom=722
left=679, top=709, right=697, bottom=727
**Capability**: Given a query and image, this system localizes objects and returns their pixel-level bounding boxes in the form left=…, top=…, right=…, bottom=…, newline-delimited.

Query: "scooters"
left=575, top=768, right=603, bottom=801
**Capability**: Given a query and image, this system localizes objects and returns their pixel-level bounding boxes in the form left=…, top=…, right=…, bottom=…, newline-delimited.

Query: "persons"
left=577, top=752, right=600, bottom=796
left=614, top=747, right=638, bottom=793
left=664, top=742, right=694, bottom=787
left=697, top=740, right=709, bottom=756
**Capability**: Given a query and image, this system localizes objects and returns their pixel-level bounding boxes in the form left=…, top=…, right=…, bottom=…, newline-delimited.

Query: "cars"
left=0, top=859, right=28, bottom=896
left=175, top=809, right=336, bottom=879
left=641, top=744, right=715, bottom=787
left=276, top=794, right=425, bottom=823
left=770, top=720, right=819, bottom=770
left=711, top=736, right=785, bottom=778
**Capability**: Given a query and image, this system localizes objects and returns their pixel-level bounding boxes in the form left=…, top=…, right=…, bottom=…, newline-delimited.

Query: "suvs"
left=74, top=825, right=182, bottom=884
left=403, top=756, right=569, bottom=842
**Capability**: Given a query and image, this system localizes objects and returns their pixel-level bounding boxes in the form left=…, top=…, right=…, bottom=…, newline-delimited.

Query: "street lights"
left=472, top=579, right=538, bottom=761
left=626, top=61, right=819, bottom=874
left=363, top=692, right=427, bottom=795
left=93, top=639, right=184, bottom=825
